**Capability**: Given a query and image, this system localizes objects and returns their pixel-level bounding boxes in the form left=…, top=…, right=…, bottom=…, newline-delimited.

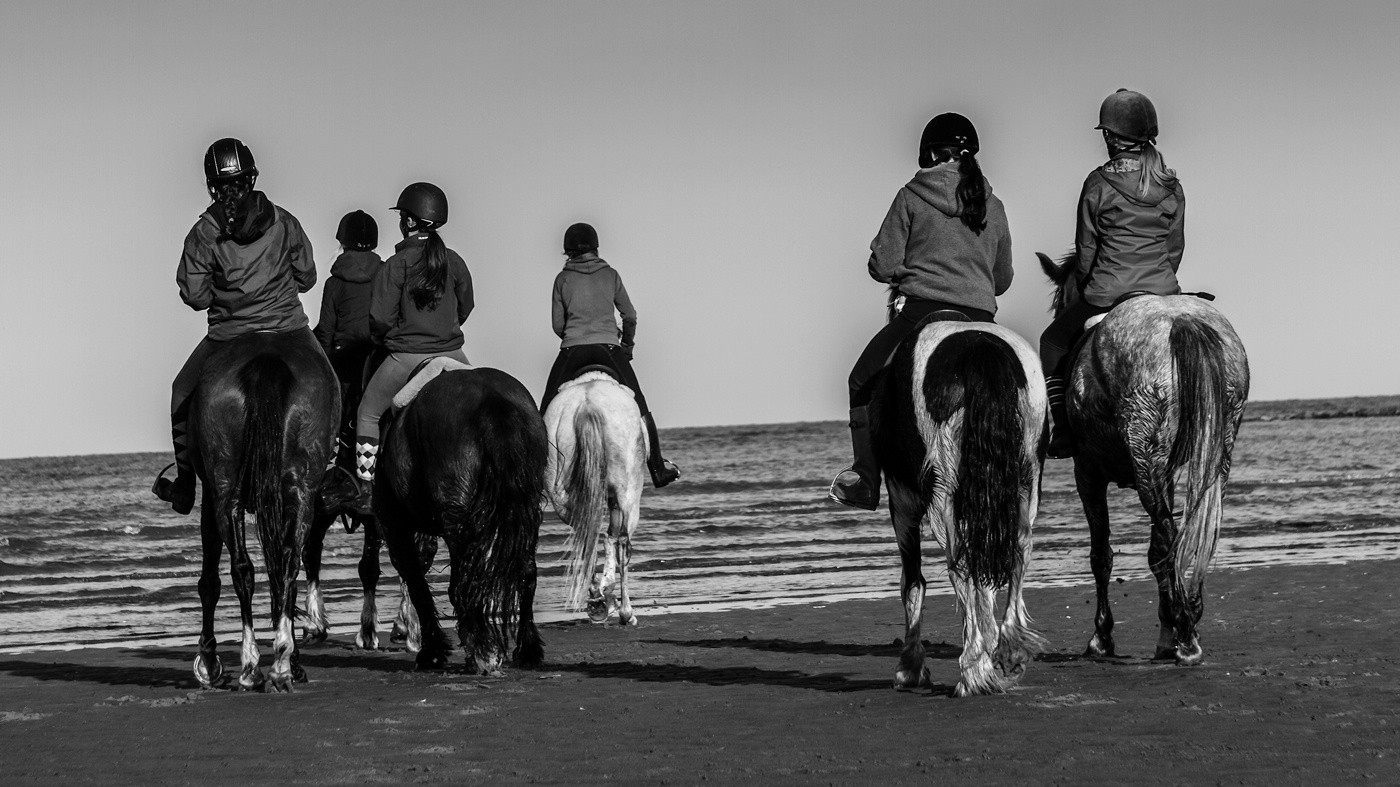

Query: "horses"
left=885, top=289, right=1053, bottom=694
left=186, top=337, right=344, bottom=698
left=541, top=372, right=651, bottom=627
left=1036, top=251, right=1251, bottom=672
left=304, top=364, right=549, bottom=673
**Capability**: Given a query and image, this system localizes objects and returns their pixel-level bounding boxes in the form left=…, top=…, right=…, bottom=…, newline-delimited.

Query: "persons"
left=155, top=137, right=357, bottom=515
left=316, top=182, right=475, bottom=517
left=540, top=223, right=680, bottom=487
left=1041, top=88, right=1186, bottom=458
left=832, top=113, right=1013, bottom=512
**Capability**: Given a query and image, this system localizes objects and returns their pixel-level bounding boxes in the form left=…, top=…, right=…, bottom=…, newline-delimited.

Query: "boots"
left=642, top=412, right=679, bottom=488
left=833, top=404, right=882, bottom=511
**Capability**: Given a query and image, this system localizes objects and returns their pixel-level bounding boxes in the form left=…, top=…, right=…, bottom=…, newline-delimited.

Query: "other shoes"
left=156, top=475, right=197, bottom=515
left=346, top=489, right=374, bottom=514
left=320, top=486, right=342, bottom=510
left=1048, top=425, right=1072, bottom=459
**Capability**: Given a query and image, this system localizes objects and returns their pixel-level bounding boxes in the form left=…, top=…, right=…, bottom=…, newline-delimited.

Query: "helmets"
left=921, top=113, right=980, bottom=151
left=563, top=223, right=599, bottom=253
left=388, top=182, right=448, bottom=229
left=336, top=210, right=378, bottom=250
left=204, top=138, right=256, bottom=180
left=1094, top=87, right=1159, bottom=142
